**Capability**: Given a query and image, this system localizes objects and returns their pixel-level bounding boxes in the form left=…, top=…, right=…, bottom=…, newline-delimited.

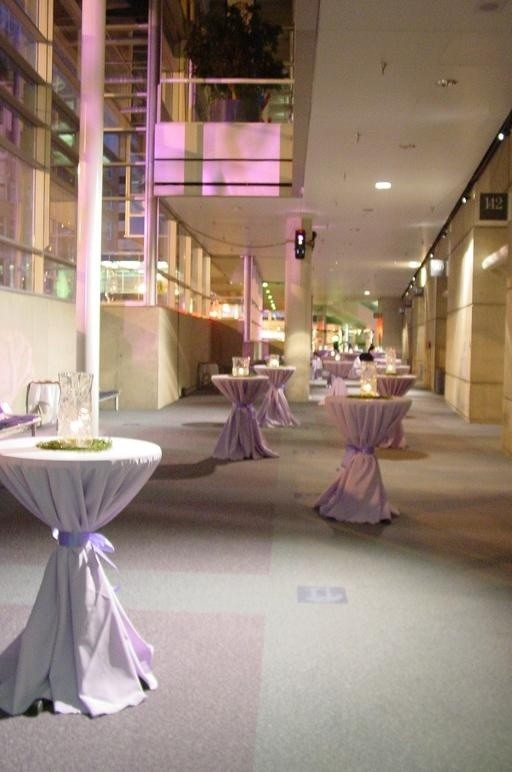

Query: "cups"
left=231, top=356, right=249, bottom=376
left=359, top=360, right=377, bottom=396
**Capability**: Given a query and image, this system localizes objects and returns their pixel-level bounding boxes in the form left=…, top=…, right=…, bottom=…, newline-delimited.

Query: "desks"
left=213, top=345, right=418, bottom=523
left=1, top=434, right=163, bottom=715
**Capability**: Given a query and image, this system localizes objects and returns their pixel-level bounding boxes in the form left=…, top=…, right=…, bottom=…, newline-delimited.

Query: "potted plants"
left=185, top=2, right=290, bottom=124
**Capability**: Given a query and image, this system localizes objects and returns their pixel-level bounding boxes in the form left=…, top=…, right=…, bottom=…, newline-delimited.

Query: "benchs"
left=98, top=388, right=120, bottom=410
left=0, top=404, right=45, bottom=437
